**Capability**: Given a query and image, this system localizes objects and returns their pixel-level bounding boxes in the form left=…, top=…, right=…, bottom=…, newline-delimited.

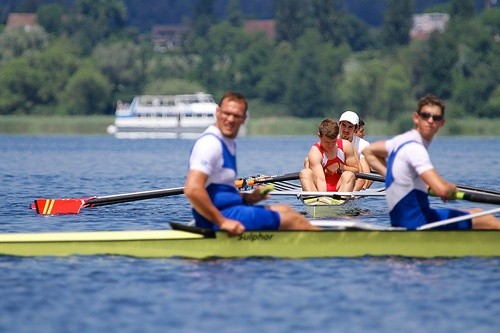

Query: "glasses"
left=419, top=112, right=442, bottom=121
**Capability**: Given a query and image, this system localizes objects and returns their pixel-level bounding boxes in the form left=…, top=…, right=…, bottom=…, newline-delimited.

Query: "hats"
left=339, top=111, right=360, bottom=127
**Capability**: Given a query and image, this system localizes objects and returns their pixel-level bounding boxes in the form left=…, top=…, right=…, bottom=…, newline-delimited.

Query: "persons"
left=183, top=92, right=320, bottom=236
left=362, top=96, right=500, bottom=230
left=304, top=111, right=374, bottom=192
left=299, top=119, right=359, bottom=200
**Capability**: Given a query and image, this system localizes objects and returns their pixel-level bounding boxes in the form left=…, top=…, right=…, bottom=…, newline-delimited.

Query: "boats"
left=107, top=92, right=247, bottom=139
left=0, top=218, right=500, bottom=258
left=296, top=195, right=367, bottom=219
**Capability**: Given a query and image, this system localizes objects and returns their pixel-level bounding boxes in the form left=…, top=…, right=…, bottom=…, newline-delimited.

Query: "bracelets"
left=338, top=162, right=340, bottom=171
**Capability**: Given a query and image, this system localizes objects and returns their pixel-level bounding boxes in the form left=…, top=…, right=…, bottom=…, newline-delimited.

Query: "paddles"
left=416, top=207, right=500, bottom=232
left=28, top=171, right=300, bottom=217
left=354, top=172, right=500, bottom=204
